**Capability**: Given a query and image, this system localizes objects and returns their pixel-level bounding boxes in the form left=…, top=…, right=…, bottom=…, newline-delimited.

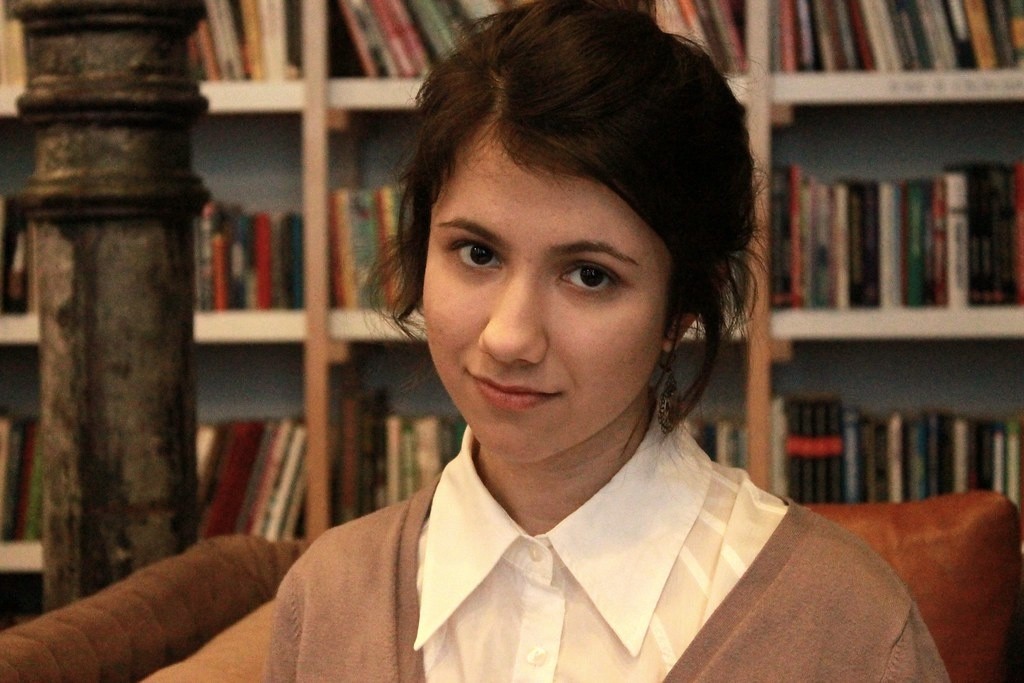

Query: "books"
left=767, top=159, right=1023, bottom=310
left=190, top=0, right=287, bottom=82
left=0, top=185, right=410, bottom=311
left=2, top=412, right=454, bottom=540
left=338, top=0, right=1024, bottom=78
left=687, top=395, right=1020, bottom=503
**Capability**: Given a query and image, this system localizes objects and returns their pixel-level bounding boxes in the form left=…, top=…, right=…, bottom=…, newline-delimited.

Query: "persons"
left=138, top=0, right=952, bottom=683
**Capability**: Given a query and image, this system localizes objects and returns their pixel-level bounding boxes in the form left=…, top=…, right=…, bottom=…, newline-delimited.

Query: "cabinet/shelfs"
left=0, top=1, right=1024, bottom=636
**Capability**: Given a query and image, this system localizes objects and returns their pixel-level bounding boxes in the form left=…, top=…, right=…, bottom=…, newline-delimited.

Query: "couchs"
left=0, top=491, right=1024, bottom=683
